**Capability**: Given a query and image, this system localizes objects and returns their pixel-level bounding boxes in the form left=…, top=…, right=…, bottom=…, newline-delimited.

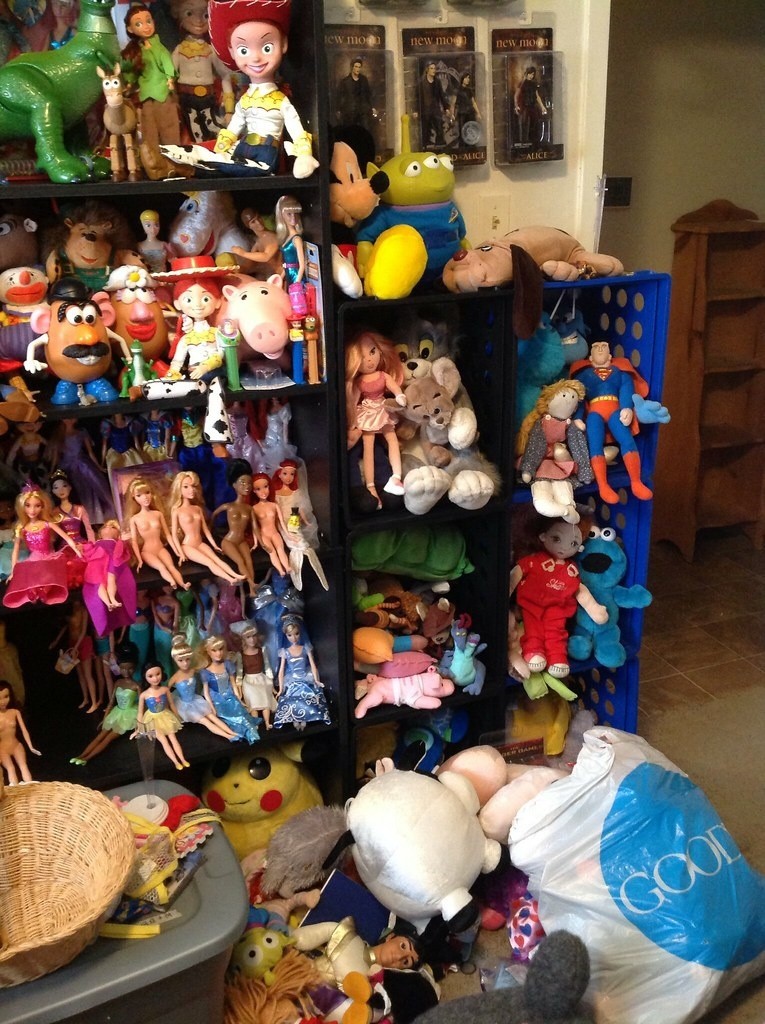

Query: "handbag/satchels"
left=507, top=725, right=764, bottom=1024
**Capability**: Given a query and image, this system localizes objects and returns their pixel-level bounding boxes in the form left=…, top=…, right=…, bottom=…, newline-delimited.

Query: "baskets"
left=0, top=767, right=135, bottom=989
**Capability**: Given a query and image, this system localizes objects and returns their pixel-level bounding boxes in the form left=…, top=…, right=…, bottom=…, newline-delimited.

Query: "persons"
left=508, top=511, right=610, bottom=676
left=343, top=330, right=409, bottom=509
left=0, top=0, right=321, bottom=190
left=0, top=397, right=335, bottom=790
left=284, top=917, right=429, bottom=1024
left=572, top=334, right=653, bottom=507
left=330, top=52, right=550, bottom=160
left=224, top=191, right=313, bottom=358
left=131, top=209, right=236, bottom=390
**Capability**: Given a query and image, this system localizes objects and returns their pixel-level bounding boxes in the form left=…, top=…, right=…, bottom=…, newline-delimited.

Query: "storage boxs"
left=1, top=779, right=249, bottom=1024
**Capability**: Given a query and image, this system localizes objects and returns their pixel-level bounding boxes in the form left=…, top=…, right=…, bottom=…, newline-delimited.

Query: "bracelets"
left=395, top=393, right=407, bottom=399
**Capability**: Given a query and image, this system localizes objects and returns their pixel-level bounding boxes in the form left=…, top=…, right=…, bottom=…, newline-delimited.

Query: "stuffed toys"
left=0, top=187, right=236, bottom=379
left=200, top=740, right=325, bottom=862
left=224, top=744, right=589, bottom=1024
left=327, top=137, right=628, bottom=297
left=341, top=293, right=655, bottom=847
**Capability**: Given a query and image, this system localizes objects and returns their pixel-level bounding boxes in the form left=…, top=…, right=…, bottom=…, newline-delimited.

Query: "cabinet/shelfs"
left=652, top=198, right=765, bottom=562
left=0, top=0, right=349, bottom=794
left=336, top=268, right=672, bottom=796
